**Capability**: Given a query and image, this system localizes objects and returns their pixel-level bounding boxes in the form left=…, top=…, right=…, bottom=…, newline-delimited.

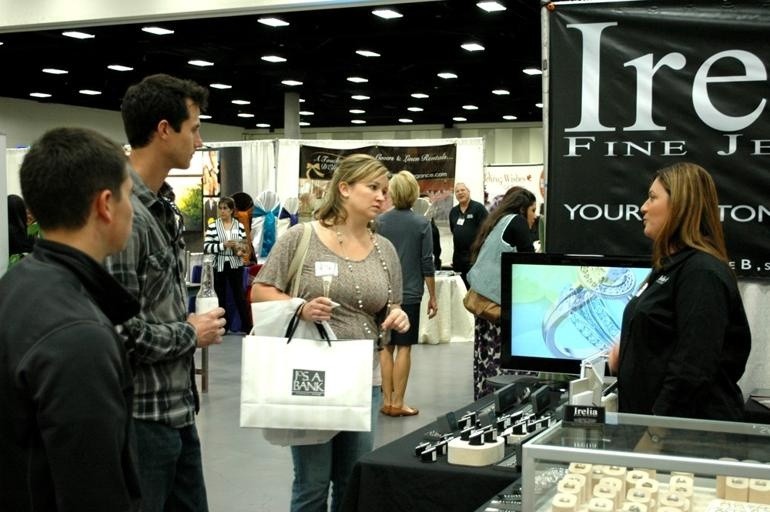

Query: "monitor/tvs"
left=500, top=253, right=659, bottom=377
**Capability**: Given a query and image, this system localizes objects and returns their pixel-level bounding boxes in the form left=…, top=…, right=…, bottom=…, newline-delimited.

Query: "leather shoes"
left=381, top=406, right=391, bottom=414
left=389, top=405, right=419, bottom=416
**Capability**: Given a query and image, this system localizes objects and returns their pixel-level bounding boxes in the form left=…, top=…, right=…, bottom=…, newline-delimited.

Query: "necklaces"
left=333, top=223, right=391, bottom=338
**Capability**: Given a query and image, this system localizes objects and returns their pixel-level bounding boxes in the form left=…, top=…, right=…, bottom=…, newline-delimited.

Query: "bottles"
left=195, top=255, right=222, bottom=344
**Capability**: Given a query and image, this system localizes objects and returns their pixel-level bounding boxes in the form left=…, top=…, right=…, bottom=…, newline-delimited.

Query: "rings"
left=560, top=463, right=767, bottom=511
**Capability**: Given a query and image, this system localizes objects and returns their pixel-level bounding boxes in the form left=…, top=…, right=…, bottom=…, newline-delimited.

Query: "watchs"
left=646, top=428, right=667, bottom=443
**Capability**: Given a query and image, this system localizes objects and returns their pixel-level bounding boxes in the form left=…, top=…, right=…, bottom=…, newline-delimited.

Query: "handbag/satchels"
left=463, top=290, right=501, bottom=321
left=239, top=302, right=374, bottom=432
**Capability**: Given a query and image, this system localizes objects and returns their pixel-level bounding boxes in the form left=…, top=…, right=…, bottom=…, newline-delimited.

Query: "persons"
left=484, top=191, right=490, bottom=211
left=250, top=154, right=411, bottom=512
left=370, top=170, right=438, bottom=417
left=538, top=203, right=544, bottom=254
left=8, top=194, right=40, bottom=265
left=449, top=183, right=489, bottom=290
left=102, top=74, right=226, bottom=512
left=608, top=162, right=753, bottom=479
left=0, top=127, right=142, bottom=512
left=420, top=194, right=442, bottom=271
left=158, top=181, right=185, bottom=255
left=204, top=192, right=263, bottom=335
left=463, top=187, right=537, bottom=401
